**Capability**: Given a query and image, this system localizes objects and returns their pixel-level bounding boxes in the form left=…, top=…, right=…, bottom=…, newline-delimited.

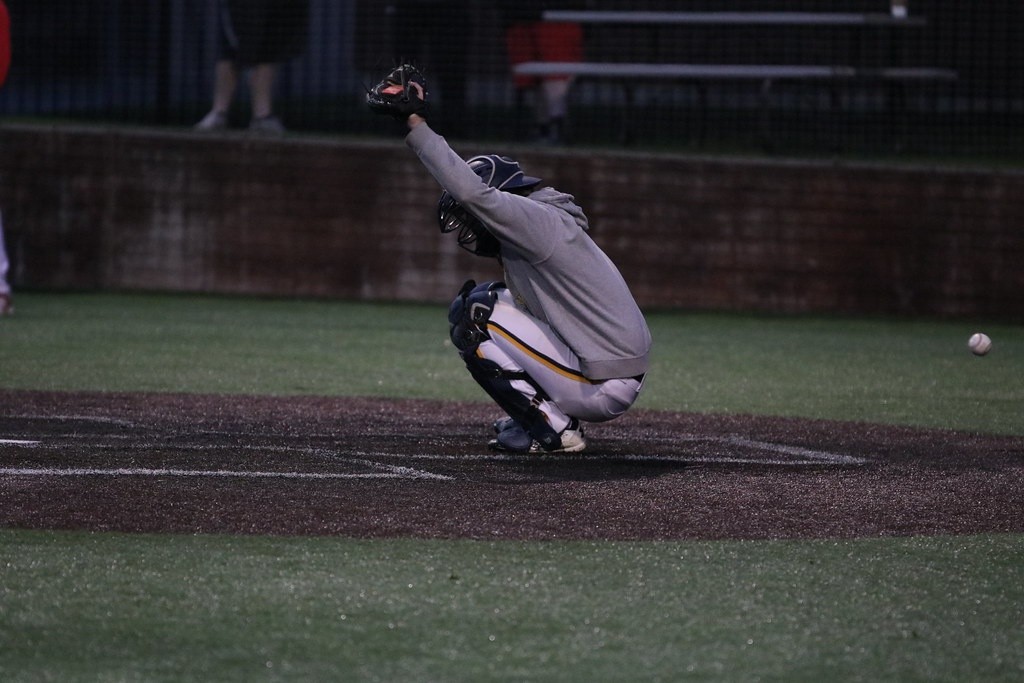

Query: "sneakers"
left=497, top=426, right=588, bottom=455
left=493, top=414, right=520, bottom=434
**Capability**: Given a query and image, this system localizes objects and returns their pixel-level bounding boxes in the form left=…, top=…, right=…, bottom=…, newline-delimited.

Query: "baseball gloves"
left=367, top=65, right=433, bottom=120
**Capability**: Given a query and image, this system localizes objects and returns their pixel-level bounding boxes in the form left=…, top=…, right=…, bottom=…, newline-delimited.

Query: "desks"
left=540, top=10, right=928, bottom=157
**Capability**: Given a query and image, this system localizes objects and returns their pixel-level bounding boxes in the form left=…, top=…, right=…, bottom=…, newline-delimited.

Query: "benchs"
left=862, top=63, right=960, bottom=82
left=510, top=59, right=876, bottom=85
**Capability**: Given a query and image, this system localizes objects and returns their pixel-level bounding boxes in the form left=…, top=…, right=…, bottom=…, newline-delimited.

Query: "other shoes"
left=196, top=106, right=246, bottom=135
left=251, top=114, right=285, bottom=136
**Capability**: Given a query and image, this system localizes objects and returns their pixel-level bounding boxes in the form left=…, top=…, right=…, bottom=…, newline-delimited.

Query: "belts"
left=632, top=373, right=647, bottom=385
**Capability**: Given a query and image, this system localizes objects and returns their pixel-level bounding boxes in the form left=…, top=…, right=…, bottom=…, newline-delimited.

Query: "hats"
left=450, top=150, right=541, bottom=212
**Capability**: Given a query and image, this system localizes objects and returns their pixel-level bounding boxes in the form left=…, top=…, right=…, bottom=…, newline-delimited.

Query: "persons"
left=366, top=65, right=652, bottom=454
left=0, top=1, right=13, bottom=318
left=503, top=0, right=584, bottom=141
left=197, top=0, right=308, bottom=135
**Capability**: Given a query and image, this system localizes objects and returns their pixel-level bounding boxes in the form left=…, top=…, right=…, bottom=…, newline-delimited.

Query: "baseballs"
left=967, top=332, right=993, bottom=356
